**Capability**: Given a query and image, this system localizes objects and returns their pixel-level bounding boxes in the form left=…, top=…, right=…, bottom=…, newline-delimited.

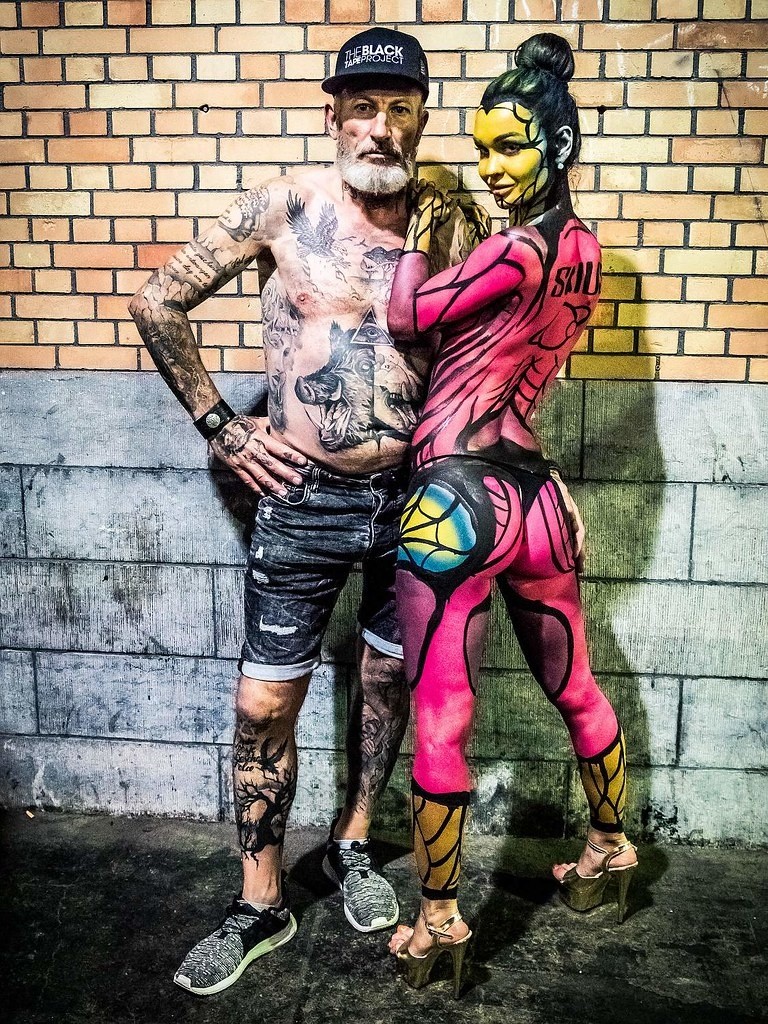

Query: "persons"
left=386, top=32, right=640, bottom=1002
left=127, top=27, right=474, bottom=995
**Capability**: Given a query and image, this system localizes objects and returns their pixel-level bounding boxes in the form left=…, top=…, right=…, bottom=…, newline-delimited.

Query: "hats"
left=321, top=27, right=429, bottom=104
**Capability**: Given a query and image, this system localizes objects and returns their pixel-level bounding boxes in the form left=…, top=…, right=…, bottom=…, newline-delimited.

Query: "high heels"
left=387, top=906, right=473, bottom=1000
left=553, top=837, right=639, bottom=924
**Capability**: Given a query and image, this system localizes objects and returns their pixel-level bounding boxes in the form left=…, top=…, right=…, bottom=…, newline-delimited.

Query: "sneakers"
left=173, top=869, right=297, bottom=995
left=322, top=807, right=399, bottom=932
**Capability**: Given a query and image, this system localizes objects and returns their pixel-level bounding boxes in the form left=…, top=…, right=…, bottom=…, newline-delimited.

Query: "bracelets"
left=193, top=399, right=237, bottom=440
